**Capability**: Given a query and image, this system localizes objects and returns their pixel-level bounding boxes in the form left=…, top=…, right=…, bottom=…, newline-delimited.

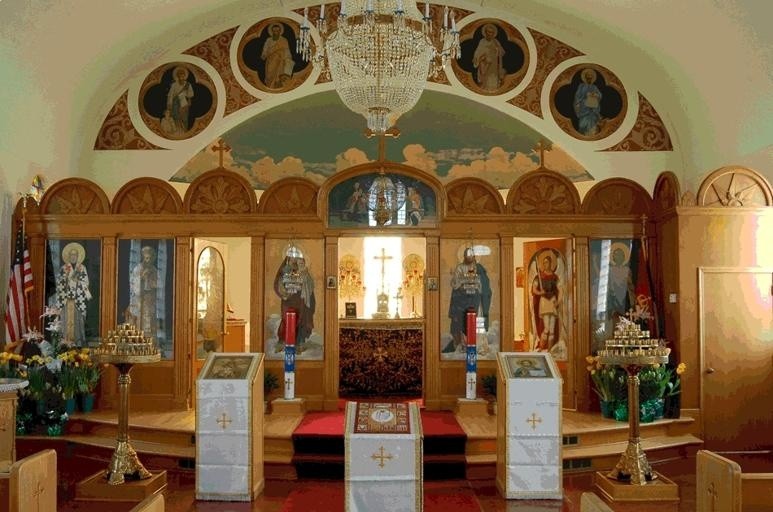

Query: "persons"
left=596, top=243, right=636, bottom=323
left=159, top=107, right=175, bottom=130
left=272, top=248, right=315, bottom=352
left=447, top=246, right=491, bottom=351
left=397, top=186, right=424, bottom=224
left=55, top=243, right=93, bottom=344
left=513, top=357, right=543, bottom=376
left=529, top=252, right=560, bottom=351
left=572, top=71, right=605, bottom=131
left=256, top=20, right=293, bottom=88
left=471, top=25, right=505, bottom=88
left=166, top=65, right=194, bottom=129
left=128, top=244, right=162, bottom=344
left=345, top=181, right=368, bottom=216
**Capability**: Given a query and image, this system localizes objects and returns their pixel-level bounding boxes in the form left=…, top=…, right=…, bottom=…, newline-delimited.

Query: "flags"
left=4, top=217, right=36, bottom=361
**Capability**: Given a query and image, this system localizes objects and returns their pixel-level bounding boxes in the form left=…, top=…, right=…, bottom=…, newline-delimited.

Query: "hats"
left=141, top=245, right=156, bottom=256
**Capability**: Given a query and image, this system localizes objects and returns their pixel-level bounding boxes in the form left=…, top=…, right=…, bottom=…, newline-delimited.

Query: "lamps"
left=295, top=0, right=461, bottom=226
